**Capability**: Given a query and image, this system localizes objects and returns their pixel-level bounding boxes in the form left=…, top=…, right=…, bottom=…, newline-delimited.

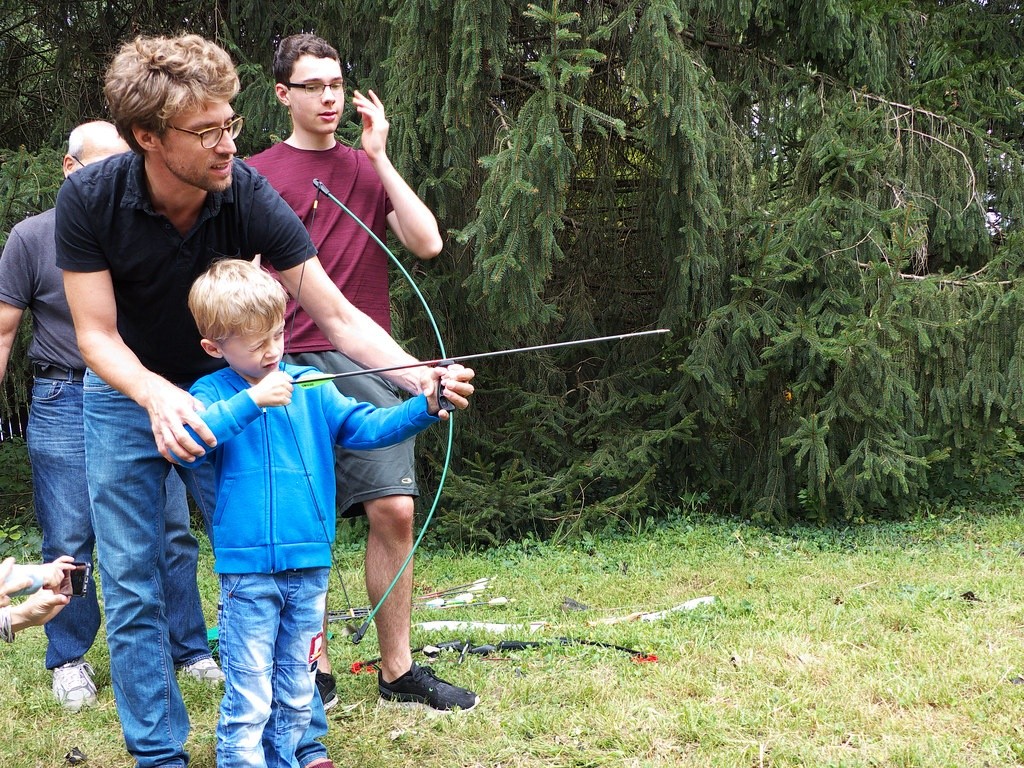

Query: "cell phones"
left=42, top=561, right=91, bottom=598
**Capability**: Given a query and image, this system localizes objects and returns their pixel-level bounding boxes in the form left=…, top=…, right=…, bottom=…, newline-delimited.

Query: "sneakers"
left=51, top=659, right=98, bottom=713
left=314, top=668, right=338, bottom=711
left=184, top=658, right=226, bottom=688
left=371, top=663, right=481, bottom=716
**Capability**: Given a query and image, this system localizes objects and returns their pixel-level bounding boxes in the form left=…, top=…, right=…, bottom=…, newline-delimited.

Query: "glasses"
left=284, top=84, right=347, bottom=98
left=166, top=113, right=245, bottom=149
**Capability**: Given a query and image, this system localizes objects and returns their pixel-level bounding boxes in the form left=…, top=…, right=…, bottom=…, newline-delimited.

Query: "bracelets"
left=0, top=605, right=15, bottom=643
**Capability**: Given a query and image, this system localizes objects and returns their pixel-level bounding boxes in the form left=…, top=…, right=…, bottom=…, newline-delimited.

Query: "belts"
left=34, top=365, right=84, bottom=382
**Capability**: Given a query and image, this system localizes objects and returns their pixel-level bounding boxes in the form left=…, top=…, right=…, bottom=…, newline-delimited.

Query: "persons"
left=169, top=258, right=454, bottom=768
left=0, top=121, right=228, bottom=714
left=54, top=36, right=474, bottom=768
left=240, top=35, right=482, bottom=713
